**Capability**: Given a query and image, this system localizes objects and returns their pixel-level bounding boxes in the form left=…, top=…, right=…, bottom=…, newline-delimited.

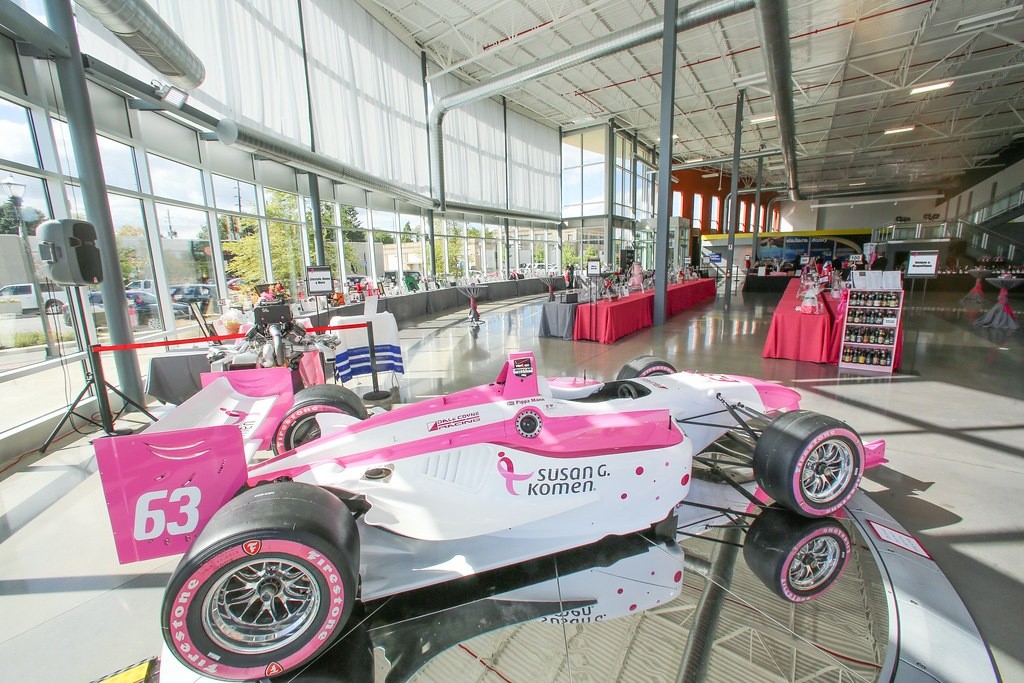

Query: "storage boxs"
left=561, top=293, right=578, bottom=303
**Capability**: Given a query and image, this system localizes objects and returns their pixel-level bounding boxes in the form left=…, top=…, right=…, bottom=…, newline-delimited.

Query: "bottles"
left=845, top=326, right=894, bottom=344
left=850, top=292, right=899, bottom=306
left=848, top=309, right=895, bottom=324
left=834, top=282, right=841, bottom=298
left=842, top=346, right=890, bottom=366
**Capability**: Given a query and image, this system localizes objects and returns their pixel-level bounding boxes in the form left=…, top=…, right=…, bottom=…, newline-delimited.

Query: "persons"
left=871, top=252, right=887, bottom=270
left=754, top=257, right=760, bottom=268
left=832, top=255, right=841, bottom=270
left=855, top=251, right=869, bottom=271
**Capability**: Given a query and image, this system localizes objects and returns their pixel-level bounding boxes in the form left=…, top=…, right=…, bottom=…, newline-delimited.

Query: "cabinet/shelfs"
left=839, top=289, right=904, bottom=373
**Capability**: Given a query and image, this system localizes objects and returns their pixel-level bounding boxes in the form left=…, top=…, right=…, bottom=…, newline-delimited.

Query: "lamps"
left=894, top=201, right=898, bottom=206
left=896, top=217, right=910, bottom=222
left=851, top=204, right=854, bottom=208
left=811, top=208, right=814, bottom=212
left=733, top=72, right=767, bottom=89
left=655, top=133, right=719, bottom=178
left=908, top=79, right=955, bottom=96
left=953, top=5, right=1023, bottom=34
left=884, top=125, right=915, bottom=135
left=748, top=111, right=776, bottom=124
left=924, top=213, right=939, bottom=220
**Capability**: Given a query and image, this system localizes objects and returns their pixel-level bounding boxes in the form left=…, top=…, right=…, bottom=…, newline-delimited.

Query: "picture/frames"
left=189, top=303, right=215, bottom=345
left=907, top=249, right=940, bottom=278
left=587, top=259, right=602, bottom=277
left=204, top=324, right=222, bottom=346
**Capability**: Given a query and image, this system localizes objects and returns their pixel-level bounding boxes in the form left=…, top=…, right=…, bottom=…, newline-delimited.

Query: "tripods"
left=39, top=286, right=159, bottom=453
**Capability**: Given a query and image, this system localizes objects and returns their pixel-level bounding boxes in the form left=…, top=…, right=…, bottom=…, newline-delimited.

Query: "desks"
left=975, top=278, right=1024, bottom=329
left=536, top=277, right=563, bottom=303
left=455, top=285, right=490, bottom=325
left=144, top=275, right=901, bottom=406
left=960, top=270, right=993, bottom=304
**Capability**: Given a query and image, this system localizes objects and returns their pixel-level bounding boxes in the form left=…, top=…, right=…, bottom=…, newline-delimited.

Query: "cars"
left=525, top=263, right=553, bottom=276
left=0, top=282, right=66, bottom=314
left=125, top=280, right=156, bottom=295
left=63, top=290, right=188, bottom=330
left=171, top=284, right=250, bottom=314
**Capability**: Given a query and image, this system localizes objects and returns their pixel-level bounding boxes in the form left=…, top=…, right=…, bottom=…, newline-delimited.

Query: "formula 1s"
left=92, top=351, right=890, bottom=682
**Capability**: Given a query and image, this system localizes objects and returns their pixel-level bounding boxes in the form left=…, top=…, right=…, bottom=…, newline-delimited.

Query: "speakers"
left=36, top=218, right=103, bottom=287
left=620, top=249, right=635, bottom=271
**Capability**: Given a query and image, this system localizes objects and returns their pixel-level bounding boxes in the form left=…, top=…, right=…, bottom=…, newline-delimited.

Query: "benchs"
left=620, top=250, right=634, bottom=271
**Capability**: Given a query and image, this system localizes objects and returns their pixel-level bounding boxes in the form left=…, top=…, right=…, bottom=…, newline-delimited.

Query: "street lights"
left=0, top=173, right=63, bottom=361
